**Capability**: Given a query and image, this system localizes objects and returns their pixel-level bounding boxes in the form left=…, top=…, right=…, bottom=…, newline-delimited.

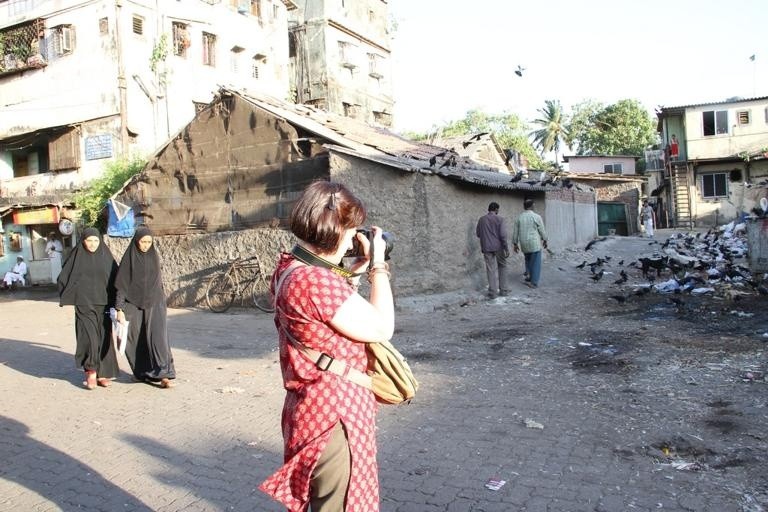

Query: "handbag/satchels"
left=366, top=341, right=419, bottom=406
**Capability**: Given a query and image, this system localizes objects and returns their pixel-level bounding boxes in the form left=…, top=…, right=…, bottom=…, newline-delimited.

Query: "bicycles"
left=206, top=254, right=275, bottom=313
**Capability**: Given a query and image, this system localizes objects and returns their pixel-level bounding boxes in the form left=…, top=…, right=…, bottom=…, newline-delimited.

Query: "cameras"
left=343, top=228, right=394, bottom=260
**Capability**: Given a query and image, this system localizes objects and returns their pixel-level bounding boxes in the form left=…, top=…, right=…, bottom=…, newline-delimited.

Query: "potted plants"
left=10, top=44, right=29, bottom=69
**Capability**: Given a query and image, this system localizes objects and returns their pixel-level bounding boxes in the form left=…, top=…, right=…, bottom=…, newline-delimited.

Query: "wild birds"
left=461, top=132, right=489, bottom=150
left=427, top=147, right=459, bottom=171
left=615, top=270, right=629, bottom=285
left=514, top=66, right=526, bottom=77
left=576, top=255, right=612, bottom=283
left=618, top=260, right=624, bottom=264
left=608, top=293, right=631, bottom=304
left=508, top=169, right=572, bottom=189
left=503, top=148, right=514, bottom=166
left=628, top=261, right=636, bottom=266
left=634, top=229, right=768, bottom=308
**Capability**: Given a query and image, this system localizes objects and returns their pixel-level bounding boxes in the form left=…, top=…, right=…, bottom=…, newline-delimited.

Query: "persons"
left=511, top=197, right=549, bottom=289
left=55, top=227, right=121, bottom=391
left=670, top=134, right=680, bottom=162
left=475, top=201, right=513, bottom=300
left=639, top=198, right=655, bottom=240
left=255, top=177, right=397, bottom=511
left=111, top=228, right=177, bottom=389
left=0, top=254, right=28, bottom=292
left=44, top=231, right=65, bottom=293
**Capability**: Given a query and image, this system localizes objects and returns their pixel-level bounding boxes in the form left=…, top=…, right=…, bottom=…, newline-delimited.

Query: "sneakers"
left=85, top=371, right=111, bottom=388
left=161, top=377, right=169, bottom=387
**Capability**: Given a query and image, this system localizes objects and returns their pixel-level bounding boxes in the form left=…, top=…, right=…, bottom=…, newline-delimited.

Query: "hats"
left=16, top=254, right=24, bottom=260
left=49, top=231, right=56, bottom=235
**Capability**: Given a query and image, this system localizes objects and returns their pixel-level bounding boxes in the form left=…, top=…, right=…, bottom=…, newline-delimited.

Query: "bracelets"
left=368, top=260, right=390, bottom=272
left=367, top=267, right=392, bottom=284
